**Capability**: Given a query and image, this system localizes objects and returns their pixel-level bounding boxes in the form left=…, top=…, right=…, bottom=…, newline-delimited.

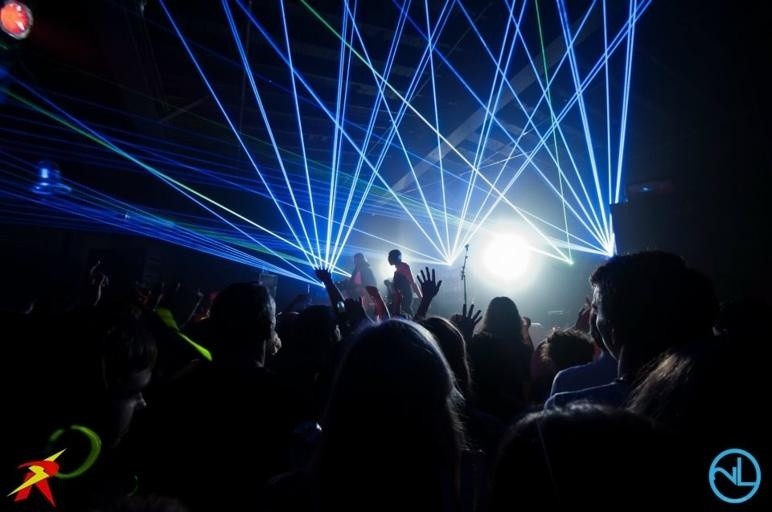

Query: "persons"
left=0, top=229, right=772, bottom=512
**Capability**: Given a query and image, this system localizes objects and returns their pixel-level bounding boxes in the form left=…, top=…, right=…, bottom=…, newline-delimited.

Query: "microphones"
left=465, top=244, right=469, bottom=251
